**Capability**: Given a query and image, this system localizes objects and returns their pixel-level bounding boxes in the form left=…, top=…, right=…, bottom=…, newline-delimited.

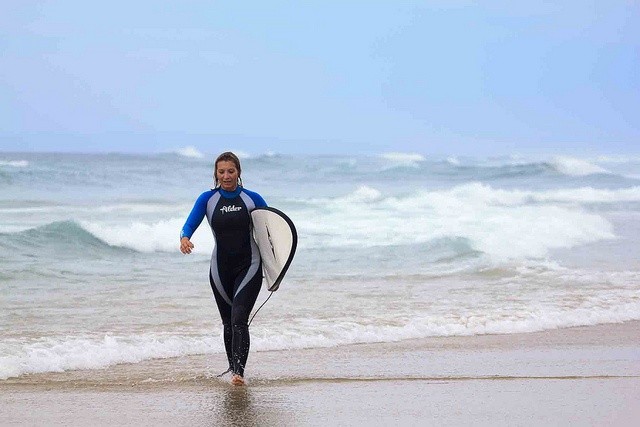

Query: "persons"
left=179, top=150, right=279, bottom=385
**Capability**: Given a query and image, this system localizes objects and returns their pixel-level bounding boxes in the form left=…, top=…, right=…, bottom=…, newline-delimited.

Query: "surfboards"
left=251, top=207, right=298, bottom=291
